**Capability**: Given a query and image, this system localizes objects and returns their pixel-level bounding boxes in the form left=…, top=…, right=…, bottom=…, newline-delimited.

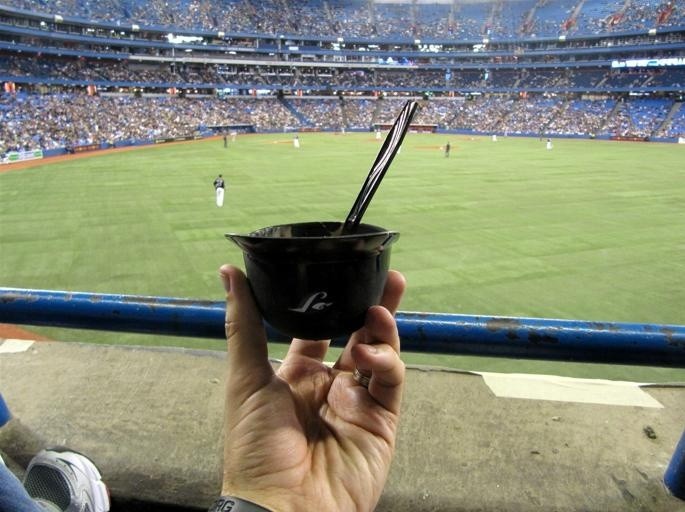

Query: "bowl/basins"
left=226, top=220, right=403, bottom=340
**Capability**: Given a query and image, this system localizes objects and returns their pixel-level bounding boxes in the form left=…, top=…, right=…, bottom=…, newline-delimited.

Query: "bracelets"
left=209, top=496, right=272, bottom=512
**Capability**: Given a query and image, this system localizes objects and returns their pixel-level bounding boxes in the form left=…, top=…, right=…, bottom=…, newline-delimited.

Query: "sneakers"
left=23, top=445, right=109, bottom=512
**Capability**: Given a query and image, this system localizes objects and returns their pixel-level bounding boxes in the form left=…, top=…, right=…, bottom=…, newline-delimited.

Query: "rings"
left=352, top=367, right=369, bottom=386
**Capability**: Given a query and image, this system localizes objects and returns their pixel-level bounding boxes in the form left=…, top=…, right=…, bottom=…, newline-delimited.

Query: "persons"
left=1, top=0, right=684, bottom=148
left=212, top=265, right=405, bottom=512
left=445, top=142, right=449, bottom=155
left=662, top=428, right=685, bottom=503
left=0, top=394, right=111, bottom=512
left=214, top=176, right=225, bottom=206
